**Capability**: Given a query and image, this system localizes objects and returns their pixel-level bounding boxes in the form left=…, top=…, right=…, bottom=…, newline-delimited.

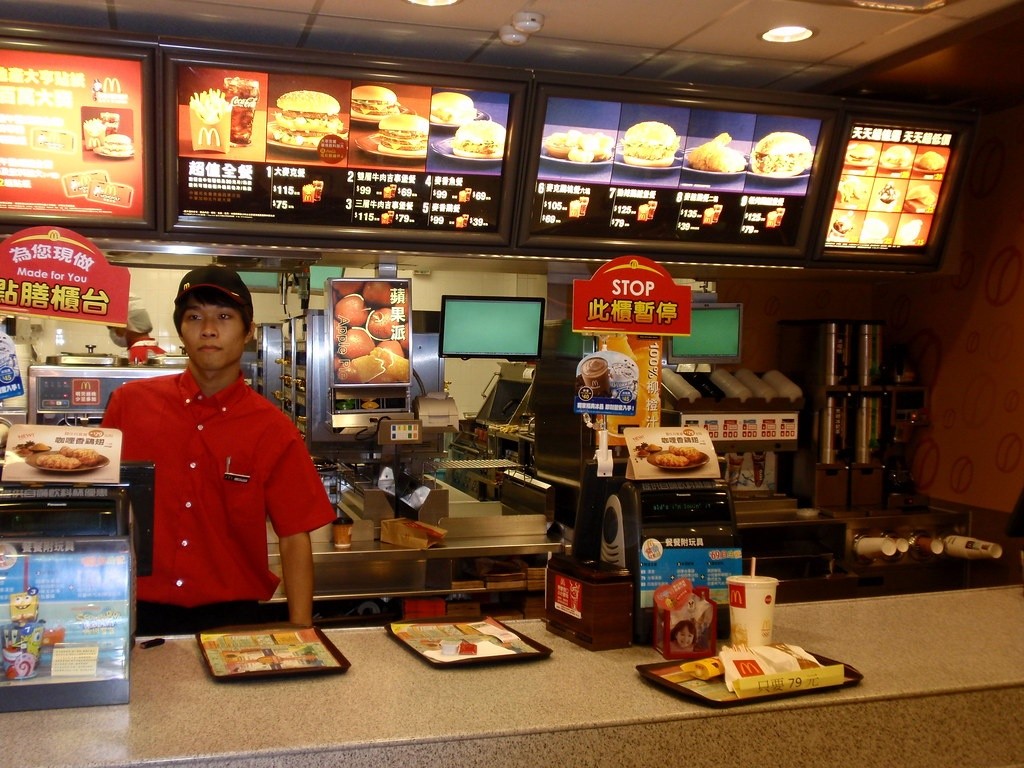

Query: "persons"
left=1006, top=487, right=1024, bottom=537
left=104, top=295, right=167, bottom=365
left=670, top=620, right=697, bottom=651
left=30, top=323, right=45, bottom=363
left=96, top=266, right=338, bottom=635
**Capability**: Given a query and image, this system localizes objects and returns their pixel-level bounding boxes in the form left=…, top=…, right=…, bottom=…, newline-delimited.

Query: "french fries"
left=189, top=87, right=233, bottom=124
left=83, top=118, right=104, bottom=135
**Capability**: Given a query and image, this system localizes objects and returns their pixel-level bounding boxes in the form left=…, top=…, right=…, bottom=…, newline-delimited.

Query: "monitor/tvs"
left=437, top=294, right=545, bottom=361
left=4, top=460, right=154, bottom=576
left=570, top=456, right=729, bottom=562
left=667, top=303, right=743, bottom=364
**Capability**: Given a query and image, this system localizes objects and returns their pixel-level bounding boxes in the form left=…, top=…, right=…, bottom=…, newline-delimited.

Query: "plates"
left=25, top=452, right=110, bottom=473
left=844, top=152, right=946, bottom=177
left=29, top=447, right=52, bottom=454
left=267, top=108, right=811, bottom=179
left=94, top=146, right=133, bottom=160
left=646, top=450, right=709, bottom=469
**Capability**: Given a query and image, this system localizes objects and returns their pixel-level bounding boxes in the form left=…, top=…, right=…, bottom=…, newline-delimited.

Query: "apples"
left=331, top=280, right=408, bottom=364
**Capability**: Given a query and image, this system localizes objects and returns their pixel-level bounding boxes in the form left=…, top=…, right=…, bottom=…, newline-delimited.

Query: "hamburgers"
left=847, top=143, right=876, bottom=166
left=269, top=90, right=347, bottom=148
left=882, top=145, right=912, bottom=168
left=430, top=91, right=507, bottom=159
left=350, top=86, right=430, bottom=157
left=103, top=134, right=131, bottom=157
left=621, top=120, right=680, bottom=168
left=750, top=131, right=814, bottom=178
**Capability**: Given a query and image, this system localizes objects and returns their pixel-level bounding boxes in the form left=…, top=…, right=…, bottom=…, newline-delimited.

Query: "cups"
left=711, top=369, right=803, bottom=402
left=647, top=200, right=657, bottom=219
left=661, top=368, right=700, bottom=403
left=727, top=574, right=778, bottom=649
left=331, top=517, right=354, bottom=550
left=313, top=181, right=323, bottom=201
left=462, top=214, right=469, bottom=227
left=388, top=211, right=394, bottom=224
left=581, top=358, right=612, bottom=399
left=579, top=196, right=590, bottom=217
left=100, top=113, right=119, bottom=137
left=712, top=205, right=723, bottom=224
left=464, top=188, right=471, bottom=202
left=0, top=623, right=45, bottom=681
left=389, top=185, right=397, bottom=198
left=223, top=77, right=259, bottom=147
left=776, top=208, right=786, bottom=226
left=855, top=533, right=1003, bottom=561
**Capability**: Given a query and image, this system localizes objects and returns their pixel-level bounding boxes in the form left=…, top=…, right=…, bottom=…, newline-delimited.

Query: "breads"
left=916, top=151, right=945, bottom=172
left=907, top=184, right=935, bottom=206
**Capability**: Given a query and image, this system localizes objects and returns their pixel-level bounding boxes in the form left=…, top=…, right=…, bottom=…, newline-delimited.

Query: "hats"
left=173, top=267, right=254, bottom=324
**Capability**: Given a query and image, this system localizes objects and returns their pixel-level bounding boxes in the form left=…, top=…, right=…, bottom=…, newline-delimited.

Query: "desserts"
left=544, top=129, right=615, bottom=162
